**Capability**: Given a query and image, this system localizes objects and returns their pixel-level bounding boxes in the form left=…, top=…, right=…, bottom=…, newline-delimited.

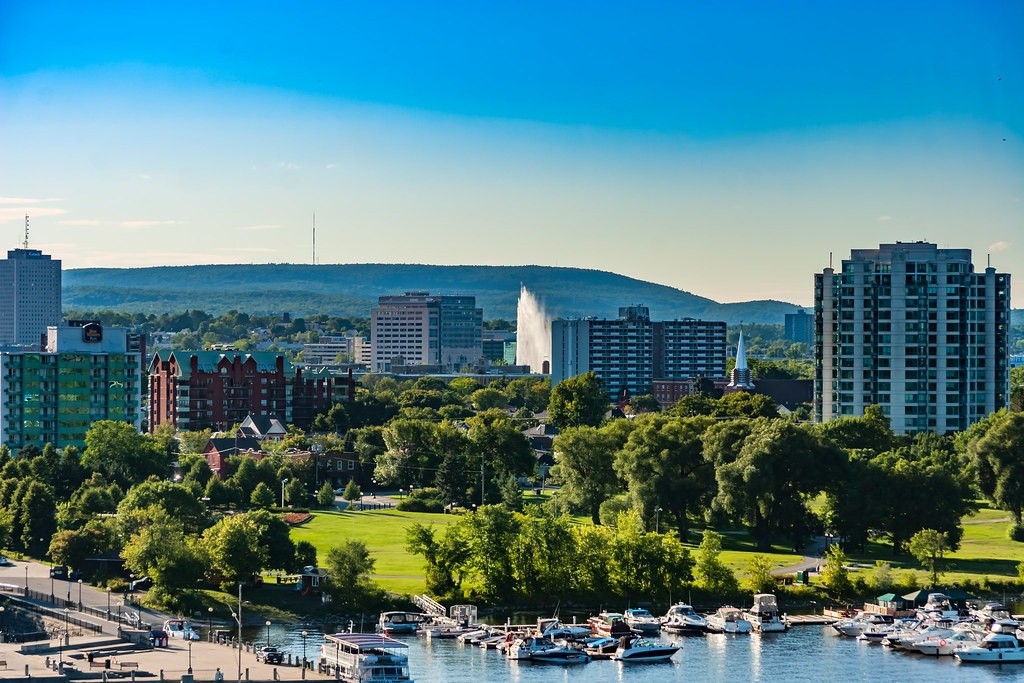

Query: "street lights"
left=208, top=606, right=214, bottom=642
left=24, top=565, right=29, bottom=597
left=57, top=634, right=64, bottom=675
left=231, top=612, right=237, bottom=647
left=50, top=572, right=55, bottom=604
left=280, top=478, right=289, bottom=508
left=399, top=488, right=404, bottom=500
left=105, top=586, right=112, bottom=621
left=77, top=579, right=84, bottom=611
left=185, top=640, right=192, bottom=674
left=300, top=631, right=308, bottom=667
left=360, top=492, right=364, bottom=510
left=264, top=620, right=272, bottom=648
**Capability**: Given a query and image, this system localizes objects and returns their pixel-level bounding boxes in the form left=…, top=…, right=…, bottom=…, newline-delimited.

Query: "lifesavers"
left=940, top=640, right=946, bottom=646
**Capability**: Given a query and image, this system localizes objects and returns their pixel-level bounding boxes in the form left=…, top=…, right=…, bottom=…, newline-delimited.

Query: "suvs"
left=256, top=647, right=283, bottom=664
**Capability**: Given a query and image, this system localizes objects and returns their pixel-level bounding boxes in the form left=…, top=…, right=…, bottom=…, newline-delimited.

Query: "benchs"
left=0, top=661, right=7, bottom=668
left=90, top=662, right=106, bottom=669
left=120, top=661, right=138, bottom=670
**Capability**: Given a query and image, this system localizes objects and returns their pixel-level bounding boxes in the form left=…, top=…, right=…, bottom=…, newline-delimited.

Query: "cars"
left=51, top=567, right=67, bottom=579
left=334, top=487, right=346, bottom=496
left=792, top=543, right=808, bottom=553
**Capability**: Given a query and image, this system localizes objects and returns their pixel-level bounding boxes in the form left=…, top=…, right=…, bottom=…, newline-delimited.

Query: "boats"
left=161, top=618, right=201, bottom=641
left=832, top=593, right=1024, bottom=663
left=377, top=593, right=791, bottom=665
left=318, top=632, right=415, bottom=683
